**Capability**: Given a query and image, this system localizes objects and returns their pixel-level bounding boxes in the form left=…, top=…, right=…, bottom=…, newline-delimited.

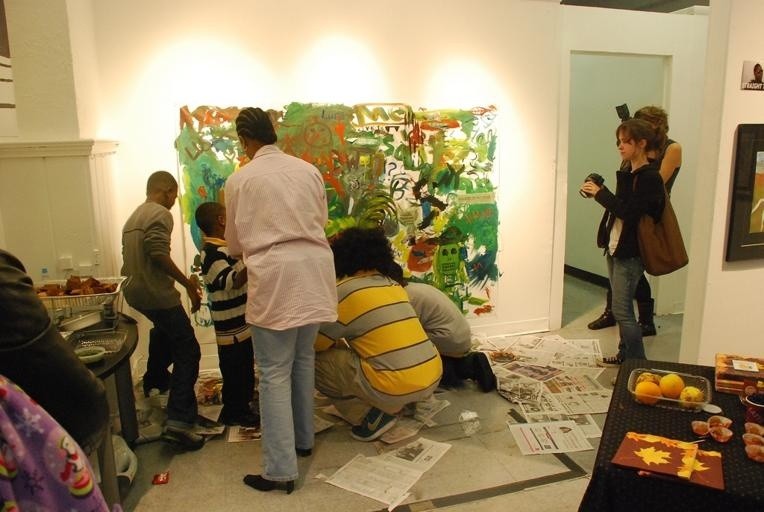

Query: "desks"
left=68, top=311, right=138, bottom=451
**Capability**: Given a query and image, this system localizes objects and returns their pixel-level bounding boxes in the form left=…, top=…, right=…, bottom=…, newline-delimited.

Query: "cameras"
left=615, top=103, right=632, bottom=147
left=579, top=172, right=605, bottom=199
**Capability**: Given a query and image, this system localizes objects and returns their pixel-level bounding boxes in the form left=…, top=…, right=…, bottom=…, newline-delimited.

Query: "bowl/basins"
left=74, top=346, right=106, bottom=364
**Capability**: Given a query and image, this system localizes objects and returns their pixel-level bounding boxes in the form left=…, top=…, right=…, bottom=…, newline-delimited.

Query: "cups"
left=690, top=415, right=764, bottom=463
left=743, top=395, right=764, bottom=425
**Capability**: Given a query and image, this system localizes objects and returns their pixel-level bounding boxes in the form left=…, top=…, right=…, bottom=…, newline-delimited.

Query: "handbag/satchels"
left=631, top=167, right=690, bottom=276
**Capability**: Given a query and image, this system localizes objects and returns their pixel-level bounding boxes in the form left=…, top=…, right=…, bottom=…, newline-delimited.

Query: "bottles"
left=41, top=266, right=50, bottom=281
left=47, top=298, right=57, bottom=329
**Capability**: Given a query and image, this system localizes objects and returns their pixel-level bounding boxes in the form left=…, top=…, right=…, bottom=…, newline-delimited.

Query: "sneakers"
left=466, top=352, right=498, bottom=393
left=161, top=427, right=205, bottom=451
left=349, top=407, right=399, bottom=442
left=144, top=372, right=172, bottom=398
left=216, top=406, right=260, bottom=427
left=597, top=354, right=624, bottom=368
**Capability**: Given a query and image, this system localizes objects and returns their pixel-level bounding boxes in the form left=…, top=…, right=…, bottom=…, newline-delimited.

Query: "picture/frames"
left=724, top=123, right=763, bottom=261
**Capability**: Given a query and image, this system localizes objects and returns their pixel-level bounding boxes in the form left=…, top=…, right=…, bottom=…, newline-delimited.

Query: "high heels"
left=243, top=474, right=295, bottom=495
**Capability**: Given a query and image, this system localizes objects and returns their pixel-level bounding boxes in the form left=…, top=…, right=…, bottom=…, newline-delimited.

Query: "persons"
left=579, top=118, right=667, bottom=386
left=587, top=101, right=684, bottom=341
left=221, top=107, right=341, bottom=497
left=117, top=168, right=206, bottom=453
left=744, top=61, right=763, bottom=90
left=312, top=221, right=444, bottom=446
left=0, top=248, right=123, bottom=512
left=192, top=201, right=262, bottom=429
left=385, top=263, right=499, bottom=394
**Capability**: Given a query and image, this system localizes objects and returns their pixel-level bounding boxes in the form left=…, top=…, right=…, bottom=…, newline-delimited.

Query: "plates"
left=58, top=312, right=102, bottom=332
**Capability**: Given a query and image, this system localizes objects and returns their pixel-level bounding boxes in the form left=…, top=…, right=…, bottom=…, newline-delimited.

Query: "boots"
left=637, top=296, right=658, bottom=338
left=589, top=291, right=617, bottom=330
